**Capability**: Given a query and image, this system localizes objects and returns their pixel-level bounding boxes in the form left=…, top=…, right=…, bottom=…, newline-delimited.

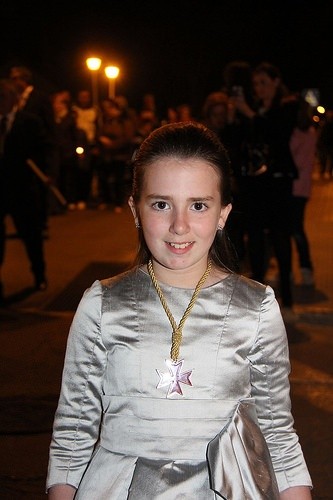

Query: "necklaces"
left=147, top=253, right=212, bottom=400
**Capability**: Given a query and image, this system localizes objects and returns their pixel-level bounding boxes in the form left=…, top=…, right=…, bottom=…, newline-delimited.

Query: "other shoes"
left=314, top=172, right=321, bottom=182
left=301, top=267, right=316, bottom=287
left=68, top=203, right=76, bottom=211
left=35, top=276, right=50, bottom=290
left=324, top=170, right=332, bottom=180
left=281, top=305, right=295, bottom=323
left=76, top=201, right=86, bottom=211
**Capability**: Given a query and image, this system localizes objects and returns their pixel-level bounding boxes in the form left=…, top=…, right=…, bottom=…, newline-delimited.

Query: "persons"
left=0, top=63, right=333, bottom=324
left=46, top=122, right=313, bottom=500
